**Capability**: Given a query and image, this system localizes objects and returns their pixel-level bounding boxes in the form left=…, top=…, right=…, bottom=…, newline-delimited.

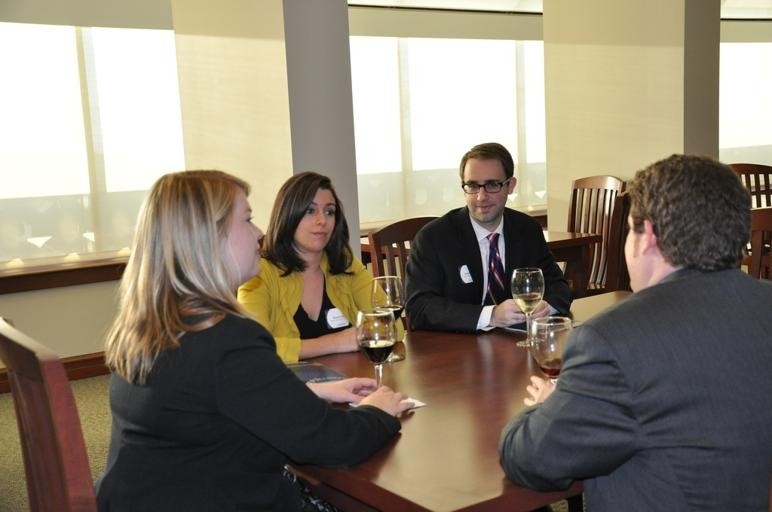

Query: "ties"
left=483, top=233, right=506, bottom=304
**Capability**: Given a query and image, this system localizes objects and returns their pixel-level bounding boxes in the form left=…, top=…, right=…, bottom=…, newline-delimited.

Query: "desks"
left=274, top=291, right=638, bottom=512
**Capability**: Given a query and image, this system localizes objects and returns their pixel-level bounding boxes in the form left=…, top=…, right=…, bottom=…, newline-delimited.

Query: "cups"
left=529, top=316, right=573, bottom=382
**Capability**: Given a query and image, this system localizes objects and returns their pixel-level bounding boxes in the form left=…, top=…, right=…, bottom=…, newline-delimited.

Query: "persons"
left=234, top=172, right=407, bottom=367
left=91, top=169, right=418, bottom=512
left=399, top=140, right=574, bottom=336
left=496, top=153, right=772, bottom=512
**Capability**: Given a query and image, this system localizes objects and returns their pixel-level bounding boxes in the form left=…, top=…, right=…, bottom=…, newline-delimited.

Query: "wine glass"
left=510, top=267, right=546, bottom=347
left=354, top=308, right=397, bottom=388
left=370, top=275, right=406, bottom=363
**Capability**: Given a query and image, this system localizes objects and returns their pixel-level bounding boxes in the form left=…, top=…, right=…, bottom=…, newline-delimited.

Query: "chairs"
left=362, top=163, right=772, bottom=313
left=0, top=315, right=98, bottom=512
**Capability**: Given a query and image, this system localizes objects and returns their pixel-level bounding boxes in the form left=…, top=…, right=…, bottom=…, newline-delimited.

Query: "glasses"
left=462, top=177, right=511, bottom=193
